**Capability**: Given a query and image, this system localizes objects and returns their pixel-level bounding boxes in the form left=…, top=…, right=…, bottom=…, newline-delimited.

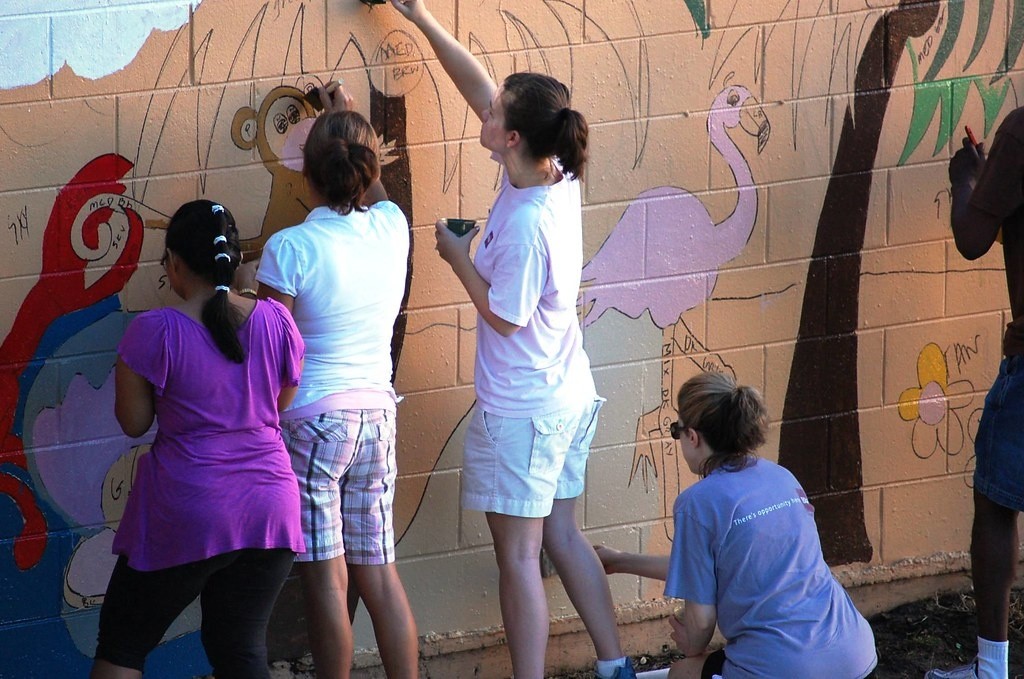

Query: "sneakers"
left=924, top=653, right=979, bottom=679
left=593, top=656, right=637, bottom=679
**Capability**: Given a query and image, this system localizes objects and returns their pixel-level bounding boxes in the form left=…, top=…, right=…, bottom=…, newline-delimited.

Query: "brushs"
left=304, top=78, right=346, bottom=113
left=360, top=0, right=391, bottom=5
left=540, top=546, right=558, bottom=579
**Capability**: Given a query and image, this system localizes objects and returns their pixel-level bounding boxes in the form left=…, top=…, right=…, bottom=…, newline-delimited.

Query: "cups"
left=448, top=218, right=476, bottom=238
left=673, top=604, right=686, bottom=624
left=238, top=242, right=263, bottom=264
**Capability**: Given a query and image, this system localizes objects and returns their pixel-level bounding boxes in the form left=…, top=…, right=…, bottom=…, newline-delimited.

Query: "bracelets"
left=239, top=288, right=257, bottom=296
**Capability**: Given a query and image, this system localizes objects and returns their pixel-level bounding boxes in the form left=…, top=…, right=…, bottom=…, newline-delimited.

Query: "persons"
left=923, top=107, right=1024, bottom=679
left=237, top=83, right=417, bottom=679
left=91, top=201, right=305, bottom=678
left=393, top=0, right=641, bottom=679
left=594, top=372, right=880, bottom=679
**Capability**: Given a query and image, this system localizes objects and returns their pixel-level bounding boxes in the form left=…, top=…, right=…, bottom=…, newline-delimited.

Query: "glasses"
left=670, top=422, right=688, bottom=439
left=159, top=255, right=168, bottom=271
